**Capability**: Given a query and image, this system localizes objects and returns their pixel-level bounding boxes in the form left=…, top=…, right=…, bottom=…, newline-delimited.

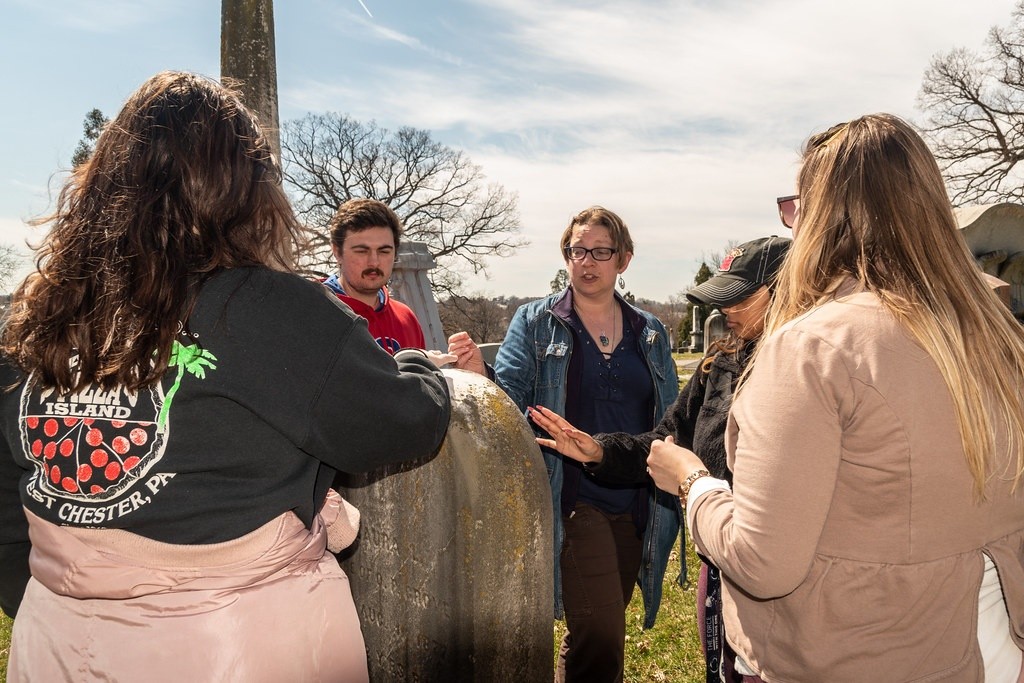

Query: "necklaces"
left=590, top=317, right=611, bottom=347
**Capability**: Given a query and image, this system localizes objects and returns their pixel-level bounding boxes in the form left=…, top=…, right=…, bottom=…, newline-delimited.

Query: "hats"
left=686, top=235, right=794, bottom=308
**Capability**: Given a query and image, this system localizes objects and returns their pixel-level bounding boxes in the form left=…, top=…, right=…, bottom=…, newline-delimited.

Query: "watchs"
left=678, top=469, right=709, bottom=510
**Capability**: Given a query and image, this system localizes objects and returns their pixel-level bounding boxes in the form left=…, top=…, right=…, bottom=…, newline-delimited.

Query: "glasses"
left=565, top=246, right=617, bottom=261
left=776, top=195, right=802, bottom=228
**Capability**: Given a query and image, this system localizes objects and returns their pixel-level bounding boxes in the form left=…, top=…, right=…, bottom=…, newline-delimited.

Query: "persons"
left=448, top=207, right=680, bottom=683
left=647, top=113, right=1024, bottom=683
left=0, top=71, right=458, bottom=683
left=527, top=235, right=793, bottom=683
left=309, top=197, right=426, bottom=358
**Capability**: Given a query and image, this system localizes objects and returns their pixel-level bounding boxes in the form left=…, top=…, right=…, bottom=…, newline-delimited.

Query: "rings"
left=646, top=466, right=649, bottom=472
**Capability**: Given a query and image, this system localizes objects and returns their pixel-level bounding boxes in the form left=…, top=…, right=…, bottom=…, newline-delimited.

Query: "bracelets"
left=392, top=347, right=428, bottom=359
